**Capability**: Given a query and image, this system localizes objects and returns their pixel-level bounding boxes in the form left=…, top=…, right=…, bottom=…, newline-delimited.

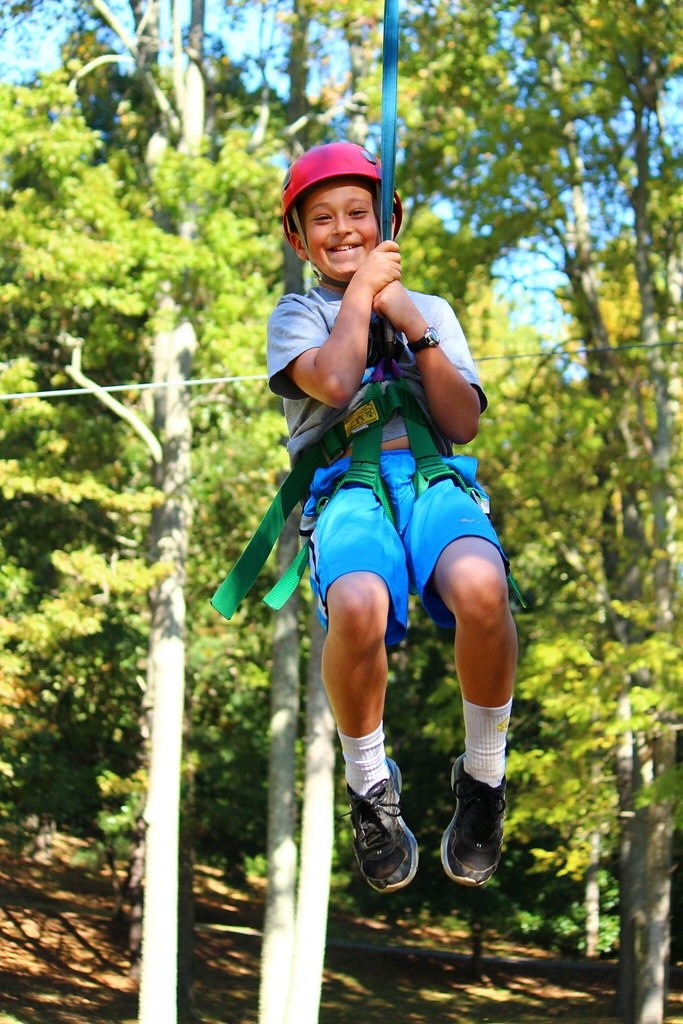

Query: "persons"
left=265, top=141, right=518, bottom=893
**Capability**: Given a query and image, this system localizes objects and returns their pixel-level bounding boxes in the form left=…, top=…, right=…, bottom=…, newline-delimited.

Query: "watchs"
left=407, top=326, right=441, bottom=353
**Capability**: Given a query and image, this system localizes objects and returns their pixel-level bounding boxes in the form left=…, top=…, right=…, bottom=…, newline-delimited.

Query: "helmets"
left=279, top=143, right=402, bottom=242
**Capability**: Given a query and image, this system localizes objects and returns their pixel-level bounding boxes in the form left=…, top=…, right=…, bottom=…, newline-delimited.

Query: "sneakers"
left=337, top=757, right=419, bottom=892
left=438, top=752, right=507, bottom=887
